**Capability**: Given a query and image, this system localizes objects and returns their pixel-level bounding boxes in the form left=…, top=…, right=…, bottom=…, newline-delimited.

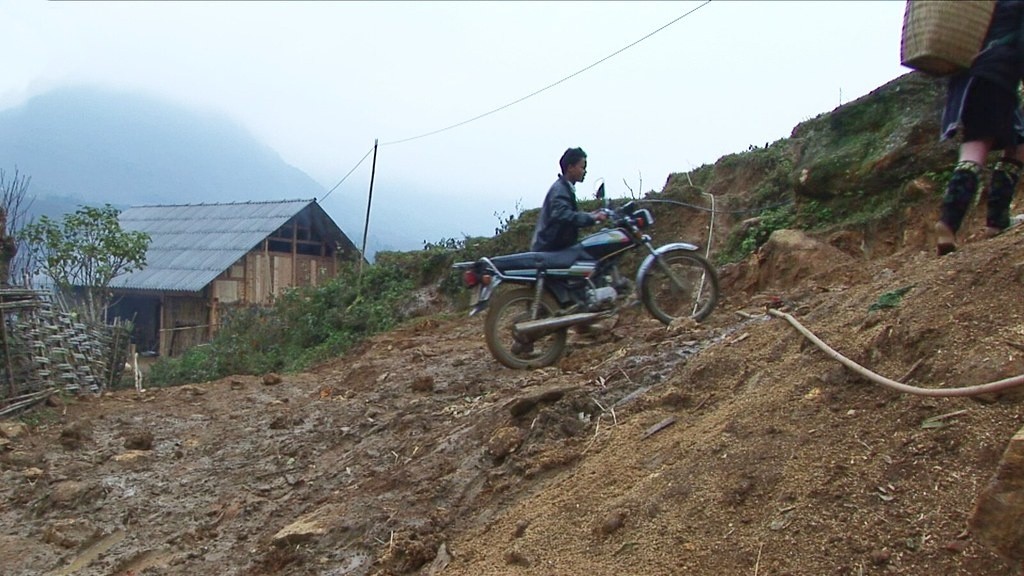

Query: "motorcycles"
left=449, top=182, right=720, bottom=371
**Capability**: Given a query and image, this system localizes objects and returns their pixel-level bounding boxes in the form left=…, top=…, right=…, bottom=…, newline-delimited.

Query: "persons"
left=935, top=1, right=1024, bottom=254
left=511, top=147, right=607, bottom=354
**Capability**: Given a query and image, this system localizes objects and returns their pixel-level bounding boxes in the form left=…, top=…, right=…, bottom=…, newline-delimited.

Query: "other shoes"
left=985, top=213, right=1024, bottom=238
left=935, top=222, right=958, bottom=255
left=575, top=324, right=603, bottom=338
left=509, top=350, right=542, bottom=360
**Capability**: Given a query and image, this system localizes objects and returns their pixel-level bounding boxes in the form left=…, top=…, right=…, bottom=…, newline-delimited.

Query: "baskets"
left=902, top=0, right=998, bottom=75
